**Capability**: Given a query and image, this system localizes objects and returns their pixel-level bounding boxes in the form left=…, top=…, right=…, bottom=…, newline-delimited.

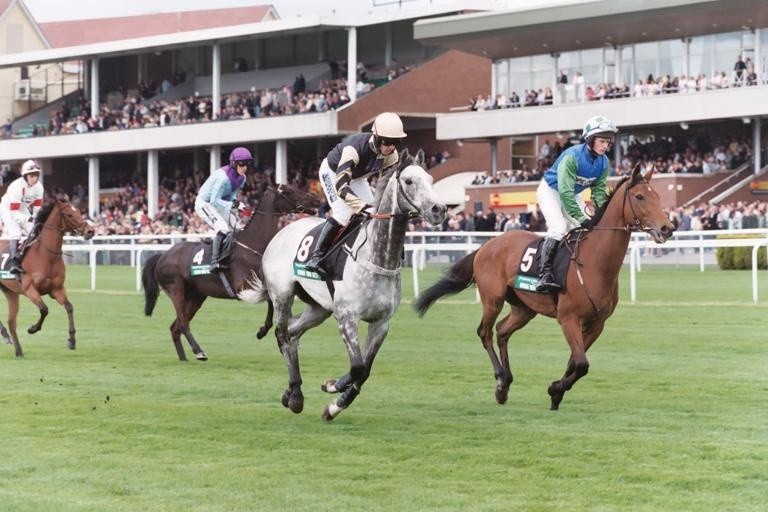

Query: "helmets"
left=229, top=147, right=252, bottom=160
left=372, top=112, right=407, bottom=138
left=582, top=115, right=618, bottom=147
left=20, top=160, right=39, bottom=175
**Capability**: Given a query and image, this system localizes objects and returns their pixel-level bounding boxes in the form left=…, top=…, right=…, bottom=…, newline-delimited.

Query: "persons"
left=0, top=159, right=44, bottom=275
left=2, top=166, right=21, bottom=185
left=6, top=56, right=411, bottom=139
left=468, top=56, right=757, bottom=112
left=605, top=133, right=768, bottom=239
left=194, top=147, right=253, bottom=273
left=536, top=116, right=619, bottom=292
left=304, top=113, right=425, bottom=275
left=405, top=134, right=586, bottom=240
left=44, top=149, right=333, bottom=240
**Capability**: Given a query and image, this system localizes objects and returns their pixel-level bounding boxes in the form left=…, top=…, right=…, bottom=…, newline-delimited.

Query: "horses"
left=0, top=195, right=95, bottom=357
left=411, top=161, right=676, bottom=411
left=142, top=170, right=321, bottom=361
left=235, top=147, right=448, bottom=422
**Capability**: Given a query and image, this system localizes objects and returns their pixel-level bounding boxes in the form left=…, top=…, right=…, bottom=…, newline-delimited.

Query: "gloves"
left=580, top=219, right=592, bottom=231
left=359, top=205, right=377, bottom=216
left=232, top=201, right=245, bottom=212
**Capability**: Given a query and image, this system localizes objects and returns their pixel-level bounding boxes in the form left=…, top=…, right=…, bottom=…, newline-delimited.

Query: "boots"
left=536, top=238, right=562, bottom=291
left=304, top=221, right=336, bottom=275
left=9, top=239, right=24, bottom=273
left=209, top=231, right=229, bottom=272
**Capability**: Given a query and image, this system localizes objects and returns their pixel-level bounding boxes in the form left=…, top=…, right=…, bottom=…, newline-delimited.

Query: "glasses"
left=582, top=122, right=615, bottom=139
left=27, top=165, right=40, bottom=169
left=380, top=138, right=400, bottom=145
left=237, top=160, right=251, bottom=166
